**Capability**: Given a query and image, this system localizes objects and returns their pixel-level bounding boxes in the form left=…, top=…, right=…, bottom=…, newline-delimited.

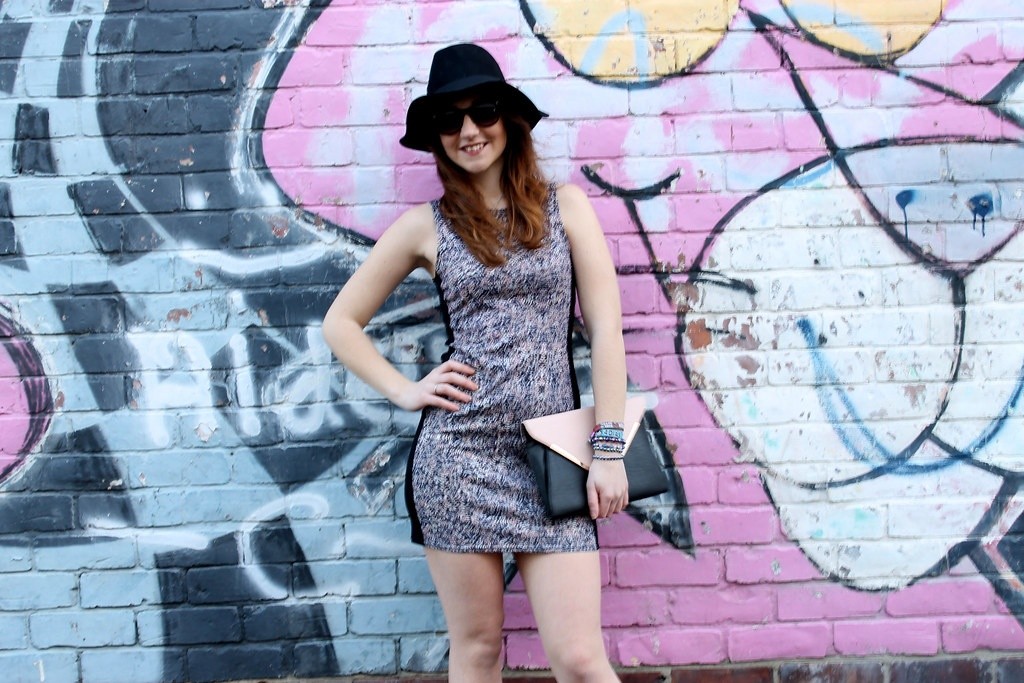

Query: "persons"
left=321, top=42, right=629, bottom=683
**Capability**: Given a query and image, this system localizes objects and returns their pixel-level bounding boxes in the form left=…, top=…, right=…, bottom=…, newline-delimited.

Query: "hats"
left=399, top=44, right=549, bottom=152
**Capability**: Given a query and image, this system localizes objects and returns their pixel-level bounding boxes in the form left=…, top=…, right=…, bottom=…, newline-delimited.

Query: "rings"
left=433, top=383, right=440, bottom=395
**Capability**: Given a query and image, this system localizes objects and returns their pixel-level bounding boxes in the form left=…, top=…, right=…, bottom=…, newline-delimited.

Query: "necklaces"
left=487, top=190, right=503, bottom=220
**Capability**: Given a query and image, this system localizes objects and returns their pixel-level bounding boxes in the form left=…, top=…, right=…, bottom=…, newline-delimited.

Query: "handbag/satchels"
left=520, top=392, right=669, bottom=521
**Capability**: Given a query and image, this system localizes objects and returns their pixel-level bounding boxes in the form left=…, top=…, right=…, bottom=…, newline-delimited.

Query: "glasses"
left=436, top=96, right=501, bottom=137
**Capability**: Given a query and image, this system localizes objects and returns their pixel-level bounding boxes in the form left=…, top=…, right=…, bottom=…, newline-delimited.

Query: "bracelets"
left=588, top=420, right=626, bottom=461
